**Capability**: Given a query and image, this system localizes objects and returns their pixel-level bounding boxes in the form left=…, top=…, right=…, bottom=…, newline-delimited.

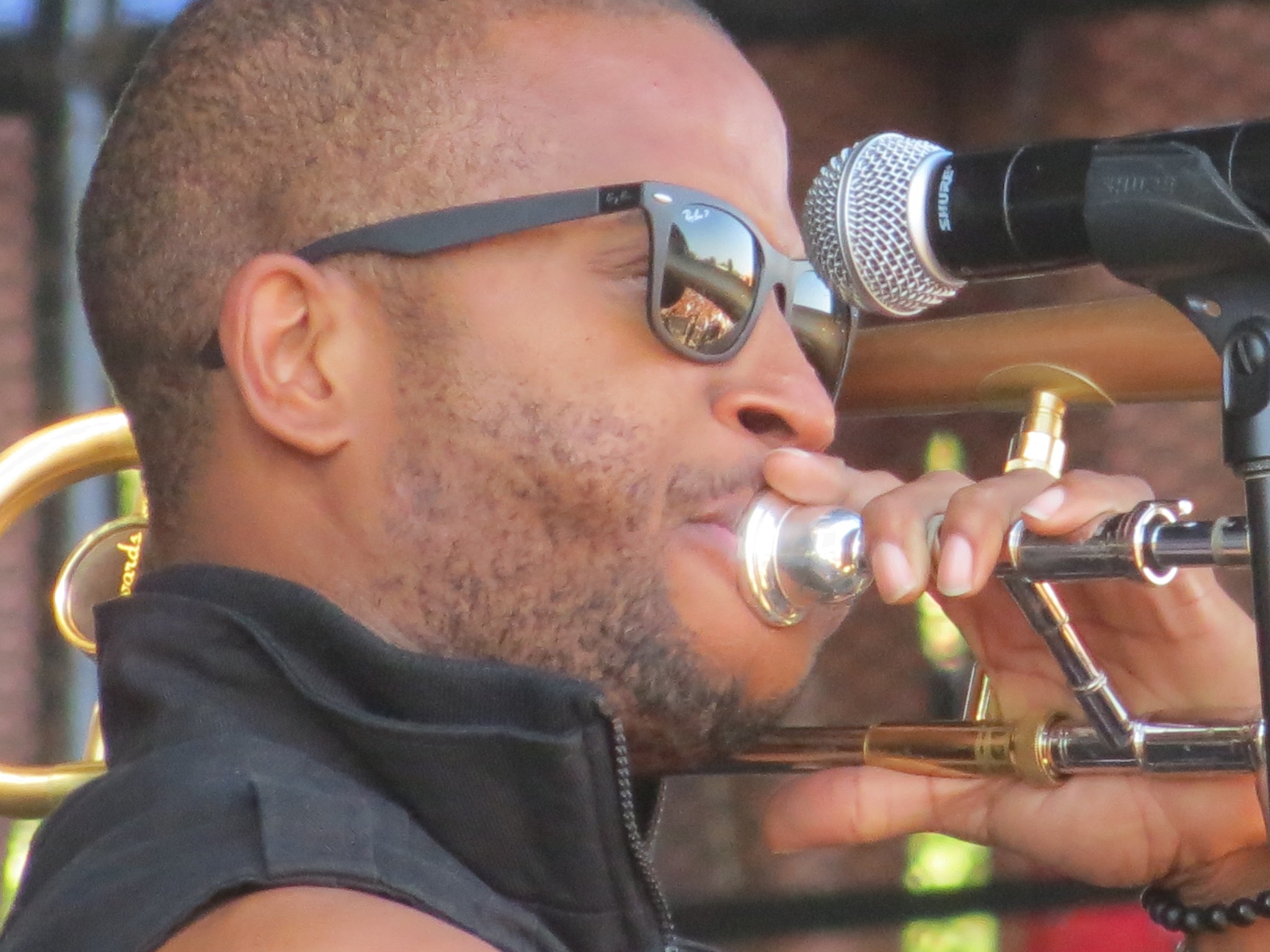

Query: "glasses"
left=202, top=181, right=861, bottom=405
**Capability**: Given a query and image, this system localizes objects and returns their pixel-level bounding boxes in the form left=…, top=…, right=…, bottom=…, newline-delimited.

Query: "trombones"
left=0, top=287, right=1267, bottom=823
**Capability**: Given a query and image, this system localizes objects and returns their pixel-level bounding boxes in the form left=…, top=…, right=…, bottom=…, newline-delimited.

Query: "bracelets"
left=1138, top=886, right=1270, bottom=934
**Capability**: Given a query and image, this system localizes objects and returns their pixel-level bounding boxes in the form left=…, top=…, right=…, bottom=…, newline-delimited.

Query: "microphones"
left=802, top=115, right=1270, bottom=319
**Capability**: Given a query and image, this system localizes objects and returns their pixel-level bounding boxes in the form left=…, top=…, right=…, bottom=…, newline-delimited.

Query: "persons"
left=0, top=0, right=1270, bottom=952
left=661, top=308, right=678, bottom=327
left=698, top=320, right=721, bottom=346
left=683, top=311, right=698, bottom=349
